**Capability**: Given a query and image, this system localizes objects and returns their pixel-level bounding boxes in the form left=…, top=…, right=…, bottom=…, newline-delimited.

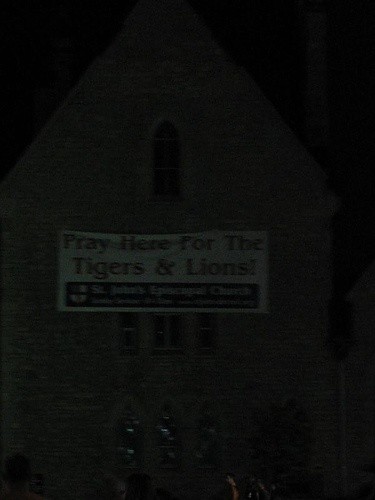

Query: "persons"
left=2, top=410, right=339, bottom=500
